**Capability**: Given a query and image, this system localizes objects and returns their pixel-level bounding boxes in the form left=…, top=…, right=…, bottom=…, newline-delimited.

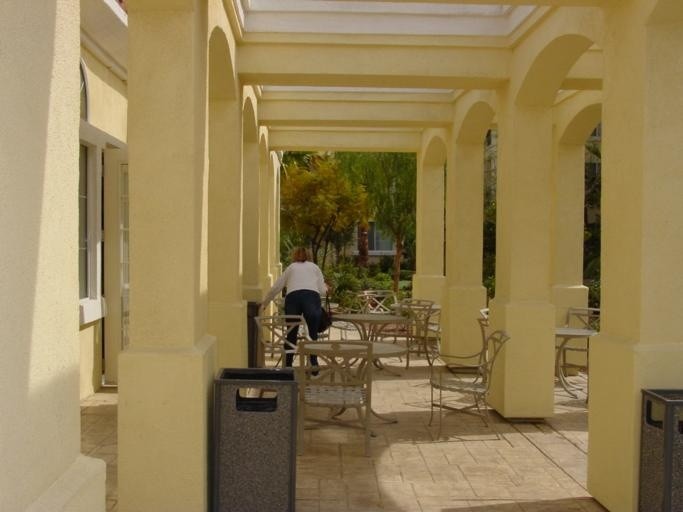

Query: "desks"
left=391, top=303, right=443, bottom=356
left=329, top=312, right=409, bottom=378
left=280, top=338, right=409, bottom=432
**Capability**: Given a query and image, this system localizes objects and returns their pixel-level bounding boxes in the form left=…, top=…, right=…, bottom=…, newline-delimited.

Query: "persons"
left=260, top=245, right=333, bottom=377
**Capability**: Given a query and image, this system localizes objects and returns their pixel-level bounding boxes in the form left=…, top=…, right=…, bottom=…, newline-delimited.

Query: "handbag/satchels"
left=317, top=295, right=334, bottom=334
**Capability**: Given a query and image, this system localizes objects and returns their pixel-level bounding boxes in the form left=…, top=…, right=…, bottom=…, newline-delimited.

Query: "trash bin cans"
left=210, top=368, right=297, bottom=512
left=248, top=302, right=261, bottom=367
left=638, top=389, right=683, bottom=512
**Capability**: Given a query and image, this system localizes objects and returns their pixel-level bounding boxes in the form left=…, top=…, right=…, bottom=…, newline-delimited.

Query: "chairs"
left=329, top=293, right=377, bottom=340
left=396, top=297, right=431, bottom=369
left=477, top=306, right=604, bottom=405
left=253, top=313, right=335, bottom=388
left=427, top=329, right=511, bottom=441
left=294, top=339, right=373, bottom=459
left=364, top=291, right=401, bottom=314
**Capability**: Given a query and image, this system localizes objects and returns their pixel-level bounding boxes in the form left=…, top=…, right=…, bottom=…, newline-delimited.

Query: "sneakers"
left=312, top=365, right=319, bottom=376
